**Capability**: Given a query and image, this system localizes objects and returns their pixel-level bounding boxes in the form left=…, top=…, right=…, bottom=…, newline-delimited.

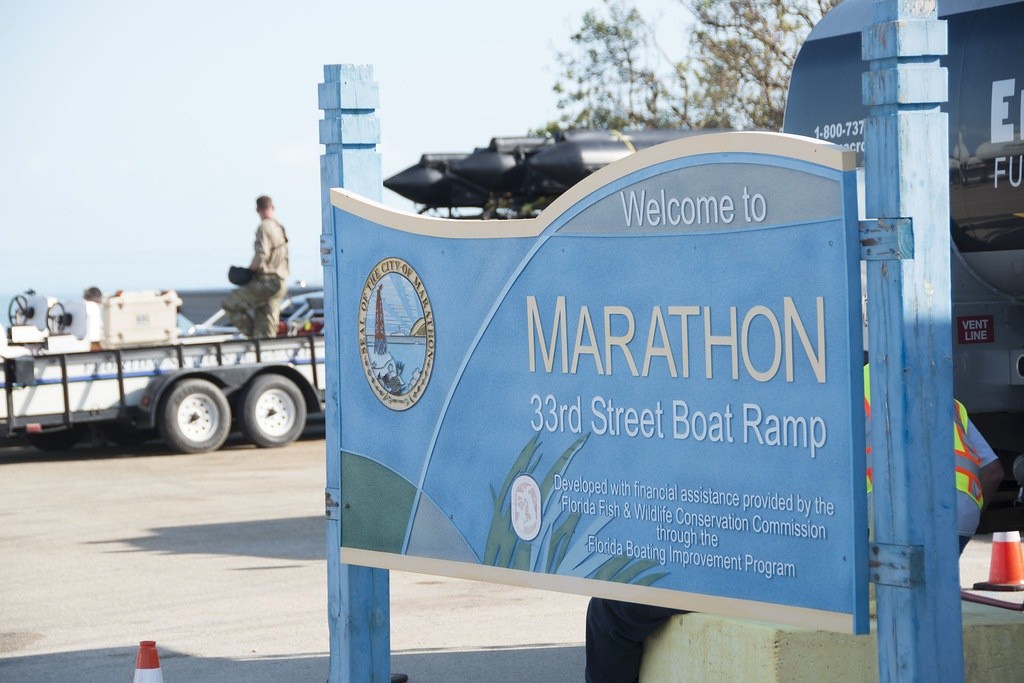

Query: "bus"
left=780, top=0, right=1024, bottom=486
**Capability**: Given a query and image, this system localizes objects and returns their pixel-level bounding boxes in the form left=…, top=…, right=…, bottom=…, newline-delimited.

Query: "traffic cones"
left=973, top=531, right=1024, bottom=591
left=134, top=641, right=163, bottom=683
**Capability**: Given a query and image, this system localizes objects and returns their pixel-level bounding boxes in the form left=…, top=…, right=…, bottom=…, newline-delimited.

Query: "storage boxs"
left=102, top=292, right=184, bottom=345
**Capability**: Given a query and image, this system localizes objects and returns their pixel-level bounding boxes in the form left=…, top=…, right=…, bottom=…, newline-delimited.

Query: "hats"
left=228, top=266, right=255, bottom=286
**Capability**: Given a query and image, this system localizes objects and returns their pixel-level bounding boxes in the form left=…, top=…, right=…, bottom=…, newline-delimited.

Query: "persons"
left=221, top=195, right=289, bottom=340
left=83, top=287, right=101, bottom=302
left=863, top=359, right=999, bottom=587
